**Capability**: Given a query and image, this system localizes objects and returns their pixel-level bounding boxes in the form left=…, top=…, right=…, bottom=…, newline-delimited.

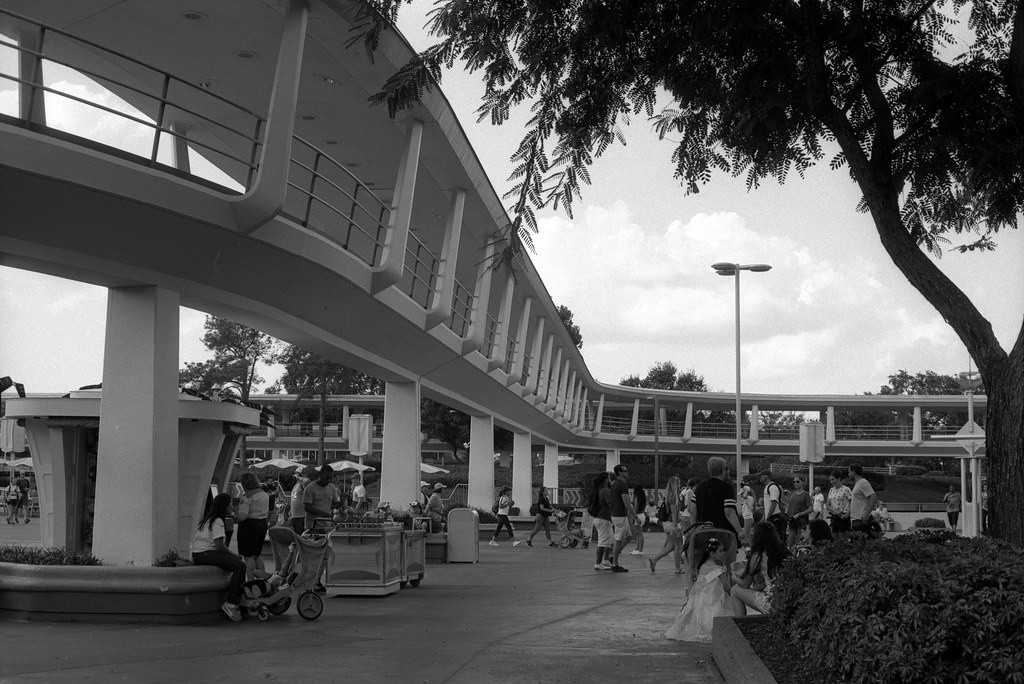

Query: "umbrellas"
left=249, top=458, right=307, bottom=482
left=421, top=463, right=450, bottom=474
left=315, top=461, right=376, bottom=505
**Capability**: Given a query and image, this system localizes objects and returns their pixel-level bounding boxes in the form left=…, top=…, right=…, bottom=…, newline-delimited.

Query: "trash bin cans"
left=446, top=508, right=481, bottom=561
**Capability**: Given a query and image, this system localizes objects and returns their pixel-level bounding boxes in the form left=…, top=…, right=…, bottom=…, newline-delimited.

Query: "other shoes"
left=525, top=539, right=532, bottom=548
left=595, top=556, right=629, bottom=573
left=549, top=541, right=559, bottom=546
left=6, top=518, right=10, bottom=524
left=15, top=518, right=19, bottom=523
left=513, top=540, right=522, bottom=547
left=630, top=550, right=643, bottom=555
left=489, top=542, right=499, bottom=547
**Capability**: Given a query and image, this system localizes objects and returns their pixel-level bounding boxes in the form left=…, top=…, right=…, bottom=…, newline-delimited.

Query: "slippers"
left=650, top=558, right=656, bottom=572
left=675, top=569, right=686, bottom=574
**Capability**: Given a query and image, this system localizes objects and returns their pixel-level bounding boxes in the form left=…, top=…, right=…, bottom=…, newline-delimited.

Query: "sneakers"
left=222, top=602, right=242, bottom=621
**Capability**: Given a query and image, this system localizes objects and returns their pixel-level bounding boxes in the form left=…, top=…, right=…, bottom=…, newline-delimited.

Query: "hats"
left=350, top=473, right=361, bottom=479
left=435, top=483, right=448, bottom=489
left=421, top=480, right=430, bottom=487
left=757, top=469, right=771, bottom=476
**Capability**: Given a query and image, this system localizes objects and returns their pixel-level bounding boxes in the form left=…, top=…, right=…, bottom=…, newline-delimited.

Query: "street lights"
left=711, top=262, right=773, bottom=520
left=646, top=395, right=660, bottom=506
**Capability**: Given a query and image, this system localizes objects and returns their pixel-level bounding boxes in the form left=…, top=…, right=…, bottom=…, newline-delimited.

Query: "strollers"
left=681, top=521, right=740, bottom=607
left=238, top=514, right=339, bottom=623
left=552, top=509, right=591, bottom=549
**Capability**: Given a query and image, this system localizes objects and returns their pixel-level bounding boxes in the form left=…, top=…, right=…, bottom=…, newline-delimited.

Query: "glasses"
left=793, top=480, right=801, bottom=484
left=323, top=472, right=334, bottom=480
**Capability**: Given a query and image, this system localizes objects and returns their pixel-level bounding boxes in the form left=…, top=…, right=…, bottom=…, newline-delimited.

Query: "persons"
left=738, top=470, right=813, bottom=548
left=944, top=485, right=988, bottom=534
left=690, top=456, right=743, bottom=550
left=645, top=476, right=701, bottom=574
left=421, top=480, right=447, bottom=522
left=730, top=522, right=794, bottom=620
left=350, top=473, right=366, bottom=511
left=192, top=471, right=269, bottom=623
left=814, top=464, right=889, bottom=533
left=5, top=470, right=30, bottom=524
left=590, top=464, right=659, bottom=573
left=663, top=538, right=731, bottom=644
left=488, top=487, right=521, bottom=547
left=526, top=487, right=559, bottom=547
left=567, top=513, right=587, bottom=539
left=262, top=477, right=281, bottom=525
left=290, top=464, right=350, bottom=592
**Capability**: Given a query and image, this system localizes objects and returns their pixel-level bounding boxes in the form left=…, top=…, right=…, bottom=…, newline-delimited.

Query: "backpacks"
left=767, top=483, right=785, bottom=511
left=657, top=498, right=670, bottom=521
left=679, top=493, right=687, bottom=512
left=492, top=495, right=507, bottom=513
